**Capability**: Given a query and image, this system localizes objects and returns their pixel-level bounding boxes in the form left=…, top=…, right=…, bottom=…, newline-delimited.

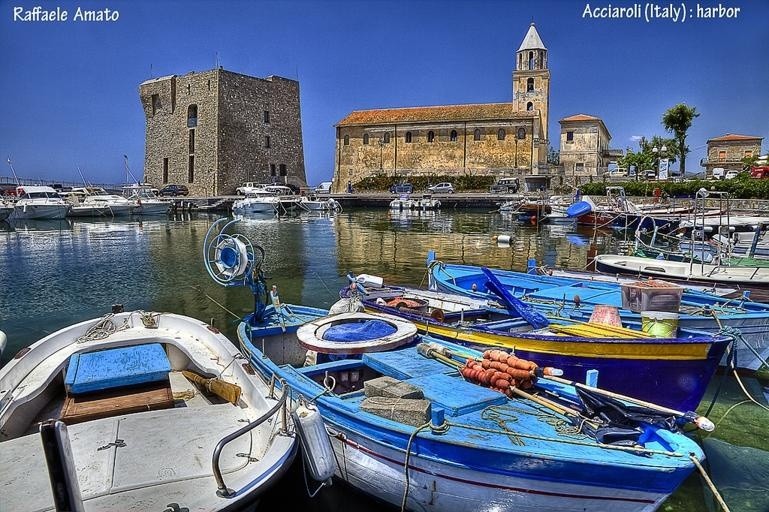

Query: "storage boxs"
left=380, top=296, right=429, bottom=312
left=620, top=278, right=684, bottom=313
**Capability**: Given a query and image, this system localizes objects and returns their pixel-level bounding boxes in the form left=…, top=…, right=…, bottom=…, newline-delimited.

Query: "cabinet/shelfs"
left=688, top=190, right=731, bottom=276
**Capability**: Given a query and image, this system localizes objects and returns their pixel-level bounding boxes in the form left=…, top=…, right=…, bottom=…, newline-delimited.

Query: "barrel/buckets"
left=640, top=310, right=680, bottom=339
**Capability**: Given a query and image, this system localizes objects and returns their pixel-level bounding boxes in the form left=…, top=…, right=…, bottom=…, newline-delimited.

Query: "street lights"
left=652, top=145, right=668, bottom=182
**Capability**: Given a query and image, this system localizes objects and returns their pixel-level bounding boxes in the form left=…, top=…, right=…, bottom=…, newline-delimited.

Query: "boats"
left=0, top=155, right=768, bottom=512
